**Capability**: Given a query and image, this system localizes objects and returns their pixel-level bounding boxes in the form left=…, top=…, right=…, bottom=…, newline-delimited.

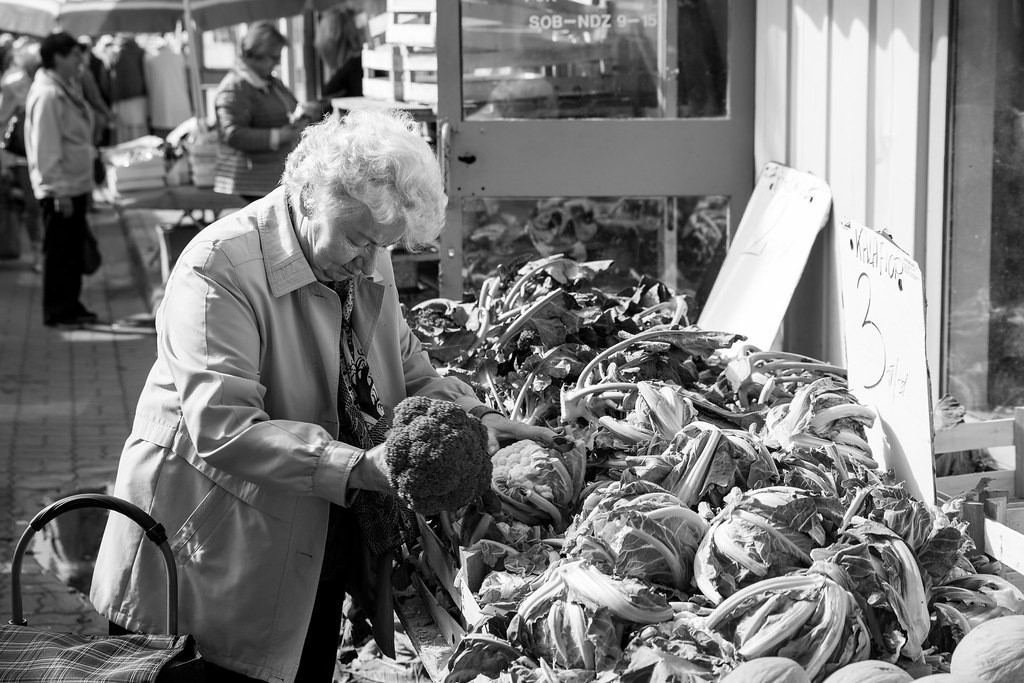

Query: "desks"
left=114, top=187, right=249, bottom=325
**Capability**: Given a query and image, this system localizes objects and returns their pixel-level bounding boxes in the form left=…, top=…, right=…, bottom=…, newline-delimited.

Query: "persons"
left=89, top=109, right=557, bottom=683
left=0, top=5, right=364, bottom=327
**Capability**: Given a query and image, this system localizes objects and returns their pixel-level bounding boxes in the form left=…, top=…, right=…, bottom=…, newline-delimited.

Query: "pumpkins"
left=722, top=614, right=1024, bottom=683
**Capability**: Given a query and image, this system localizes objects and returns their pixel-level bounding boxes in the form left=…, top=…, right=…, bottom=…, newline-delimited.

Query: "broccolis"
left=382, top=254, right=981, bottom=683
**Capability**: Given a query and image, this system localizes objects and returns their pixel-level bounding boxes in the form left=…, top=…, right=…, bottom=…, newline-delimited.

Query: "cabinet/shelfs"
left=330, top=96, right=643, bottom=277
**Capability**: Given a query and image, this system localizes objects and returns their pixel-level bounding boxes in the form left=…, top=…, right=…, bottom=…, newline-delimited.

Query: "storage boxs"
left=414, top=406, right=1024, bottom=647
left=361, top=0, right=612, bottom=101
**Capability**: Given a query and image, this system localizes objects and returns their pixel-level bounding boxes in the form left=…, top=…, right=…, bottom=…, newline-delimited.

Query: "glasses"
left=268, top=53, right=281, bottom=62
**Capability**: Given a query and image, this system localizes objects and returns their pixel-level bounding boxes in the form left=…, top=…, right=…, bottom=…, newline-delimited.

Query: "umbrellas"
left=0, top=0, right=347, bottom=119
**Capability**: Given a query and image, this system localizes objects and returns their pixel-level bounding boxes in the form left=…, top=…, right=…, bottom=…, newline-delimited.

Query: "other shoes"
left=44, top=303, right=98, bottom=329
